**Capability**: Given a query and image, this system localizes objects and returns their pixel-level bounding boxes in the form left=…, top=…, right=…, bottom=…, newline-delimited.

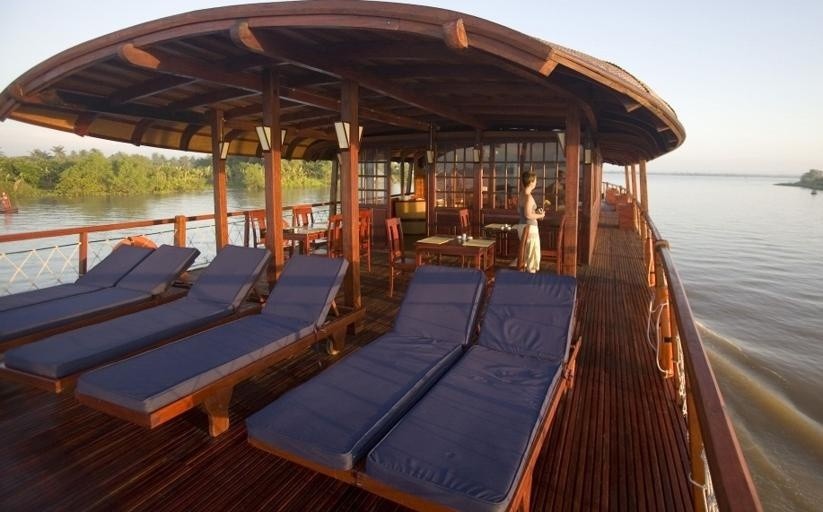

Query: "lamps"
left=282, top=128, right=287, bottom=146
left=256, top=125, right=272, bottom=153
left=473, top=149, right=481, bottom=164
left=218, top=142, right=230, bottom=160
left=359, top=125, right=364, bottom=143
left=334, top=122, right=351, bottom=151
left=584, top=148, right=592, bottom=165
left=556, top=131, right=566, bottom=157
left=427, top=150, right=434, bottom=165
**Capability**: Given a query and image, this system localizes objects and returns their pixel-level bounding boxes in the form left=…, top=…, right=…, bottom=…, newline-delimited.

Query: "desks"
left=606, top=188, right=634, bottom=230
left=395, top=198, right=427, bottom=235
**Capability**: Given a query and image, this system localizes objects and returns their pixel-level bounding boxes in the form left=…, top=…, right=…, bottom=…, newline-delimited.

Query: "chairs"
left=244, top=264, right=487, bottom=484
left=248, top=206, right=532, bottom=300
left=75, top=252, right=367, bottom=438
left=0, top=243, right=272, bottom=396
left=359, top=267, right=581, bottom=511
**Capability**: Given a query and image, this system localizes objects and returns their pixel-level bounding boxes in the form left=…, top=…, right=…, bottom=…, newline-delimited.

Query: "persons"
left=548, top=168, right=567, bottom=204
left=507, top=171, right=547, bottom=273
left=0, top=192, right=11, bottom=211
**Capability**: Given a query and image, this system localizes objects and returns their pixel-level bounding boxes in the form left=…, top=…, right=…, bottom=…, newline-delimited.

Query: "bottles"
left=462, top=232, right=466, bottom=240
